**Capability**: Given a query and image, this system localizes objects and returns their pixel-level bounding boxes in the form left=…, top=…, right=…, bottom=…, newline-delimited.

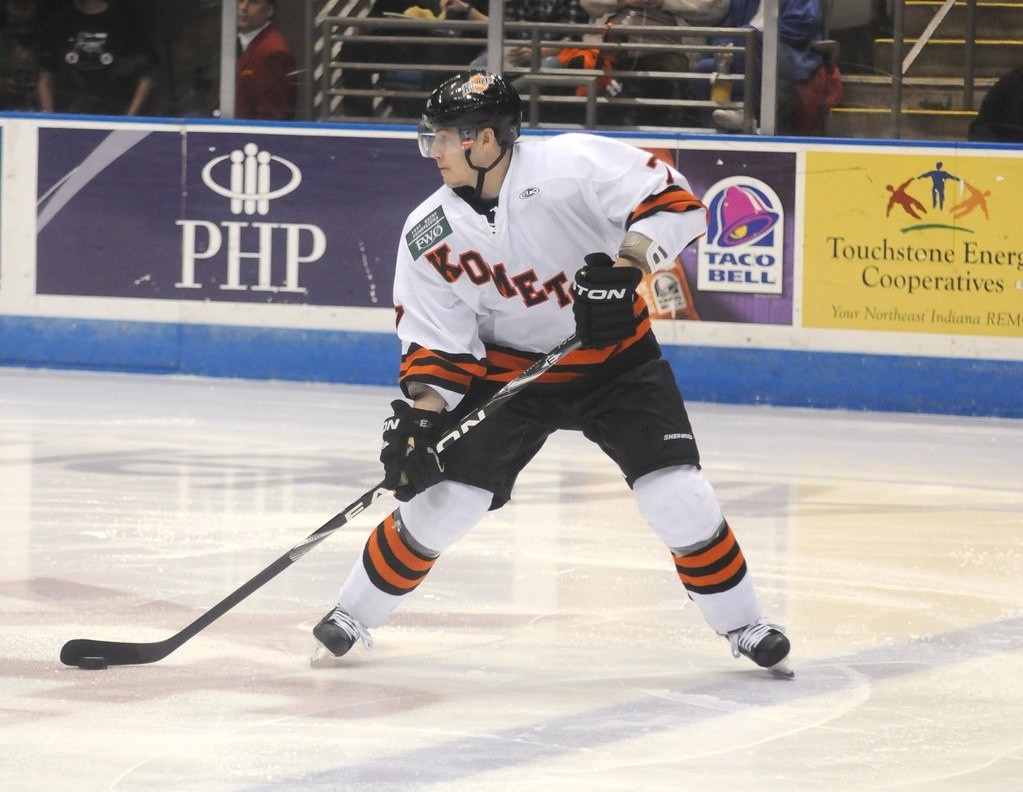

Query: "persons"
left=0, top=0, right=1023, bottom=148
left=310, top=71, right=796, bottom=680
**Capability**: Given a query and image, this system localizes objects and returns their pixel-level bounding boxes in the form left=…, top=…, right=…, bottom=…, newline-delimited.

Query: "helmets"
left=424, top=69, right=523, bottom=147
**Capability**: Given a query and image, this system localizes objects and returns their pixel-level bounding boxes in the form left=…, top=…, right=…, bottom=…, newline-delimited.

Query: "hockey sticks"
left=59, top=332, right=584, bottom=667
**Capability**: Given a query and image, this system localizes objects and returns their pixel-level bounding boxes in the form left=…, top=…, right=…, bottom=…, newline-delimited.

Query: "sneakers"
left=725, top=616, right=793, bottom=678
left=311, top=605, right=372, bottom=665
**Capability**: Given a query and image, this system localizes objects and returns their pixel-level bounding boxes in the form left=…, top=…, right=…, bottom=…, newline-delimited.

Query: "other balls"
left=78, top=656, right=108, bottom=670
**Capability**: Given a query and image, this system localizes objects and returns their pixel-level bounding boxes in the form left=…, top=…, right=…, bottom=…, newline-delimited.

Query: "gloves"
left=572, top=252, right=643, bottom=350
left=380, top=399, right=448, bottom=502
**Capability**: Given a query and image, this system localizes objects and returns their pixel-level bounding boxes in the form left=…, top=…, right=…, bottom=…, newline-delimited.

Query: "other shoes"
left=712, top=108, right=758, bottom=133
